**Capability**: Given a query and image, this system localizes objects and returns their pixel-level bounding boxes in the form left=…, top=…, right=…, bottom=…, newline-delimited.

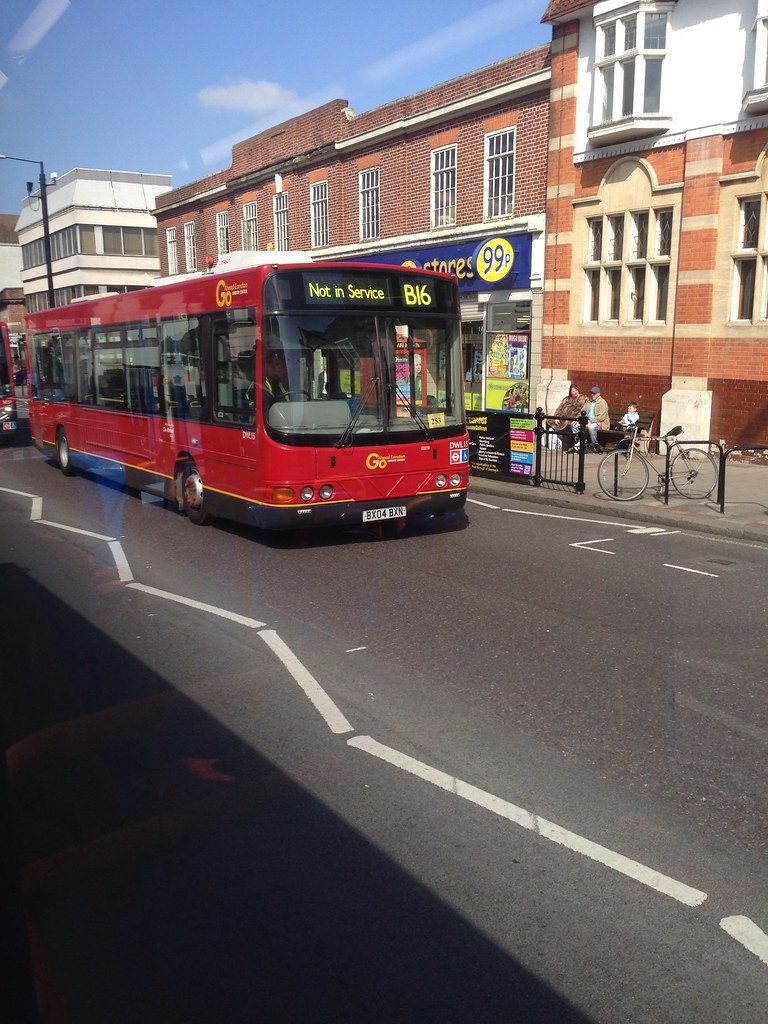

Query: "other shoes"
left=592, top=444, right=602, bottom=453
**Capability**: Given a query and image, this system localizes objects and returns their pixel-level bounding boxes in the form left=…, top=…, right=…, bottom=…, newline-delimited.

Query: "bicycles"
left=596, top=419, right=719, bottom=501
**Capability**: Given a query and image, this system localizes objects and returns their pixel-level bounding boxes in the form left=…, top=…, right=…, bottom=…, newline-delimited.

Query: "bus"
left=0, top=320, right=18, bottom=442
left=23, top=258, right=471, bottom=543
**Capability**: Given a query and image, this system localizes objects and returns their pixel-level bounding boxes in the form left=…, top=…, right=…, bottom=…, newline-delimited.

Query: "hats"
left=589, top=386, right=600, bottom=393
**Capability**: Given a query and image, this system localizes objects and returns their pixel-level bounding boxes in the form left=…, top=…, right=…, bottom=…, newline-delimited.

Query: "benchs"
left=599, top=411, right=655, bottom=454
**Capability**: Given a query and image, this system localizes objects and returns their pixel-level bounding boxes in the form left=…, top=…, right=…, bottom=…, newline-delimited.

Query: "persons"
left=245, top=355, right=291, bottom=422
left=546, top=384, right=610, bottom=453
left=614, top=401, right=639, bottom=433
